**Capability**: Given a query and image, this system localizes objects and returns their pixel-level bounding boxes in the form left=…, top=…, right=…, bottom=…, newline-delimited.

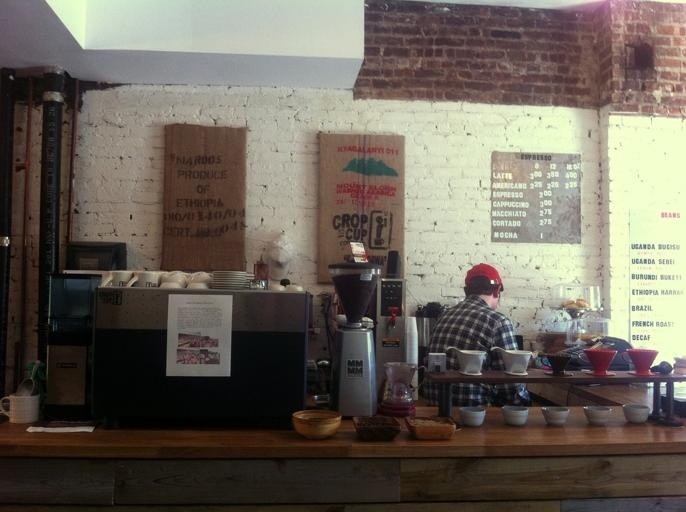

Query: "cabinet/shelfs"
left=95, top=272, right=310, bottom=429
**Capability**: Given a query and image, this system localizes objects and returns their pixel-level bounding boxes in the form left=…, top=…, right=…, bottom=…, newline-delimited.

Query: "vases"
left=564, top=319, right=587, bottom=345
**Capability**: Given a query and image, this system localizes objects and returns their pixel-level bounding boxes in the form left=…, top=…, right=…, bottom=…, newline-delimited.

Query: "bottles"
left=253, top=253, right=269, bottom=291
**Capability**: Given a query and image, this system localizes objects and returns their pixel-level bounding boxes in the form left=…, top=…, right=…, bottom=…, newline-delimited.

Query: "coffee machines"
left=327, top=260, right=382, bottom=419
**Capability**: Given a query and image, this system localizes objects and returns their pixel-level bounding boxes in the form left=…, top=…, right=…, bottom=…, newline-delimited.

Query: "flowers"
left=562, top=299, right=592, bottom=339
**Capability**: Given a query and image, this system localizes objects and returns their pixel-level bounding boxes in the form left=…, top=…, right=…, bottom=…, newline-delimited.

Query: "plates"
left=351, top=415, right=403, bottom=442
left=209, top=270, right=255, bottom=289
left=403, top=415, right=457, bottom=441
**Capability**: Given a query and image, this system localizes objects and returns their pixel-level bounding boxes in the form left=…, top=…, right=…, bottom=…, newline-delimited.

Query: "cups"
left=99, top=268, right=162, bottom=289
left=537, top=350, right=575, bottom=374
left=577, top=348, right=619, bottom=377
left=446, top=345, right=486, bottom=376
left=619, top=347, right=659, bottom=377
left=488, top=345, right=534, bottom=376
left=0, top=393, right=40, bottom=425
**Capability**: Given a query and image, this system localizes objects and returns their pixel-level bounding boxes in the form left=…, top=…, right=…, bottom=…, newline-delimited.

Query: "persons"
left=424, top=263, right=532, bottom=406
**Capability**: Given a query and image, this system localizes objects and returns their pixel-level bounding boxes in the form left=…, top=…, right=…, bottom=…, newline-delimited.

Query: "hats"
left=465, top=263, right=504, bottom=292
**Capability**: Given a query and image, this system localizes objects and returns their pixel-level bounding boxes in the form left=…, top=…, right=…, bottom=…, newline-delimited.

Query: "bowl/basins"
left=458, top=405, right=486, bottom=427
left=540, top=406, right=571, bottom=426
left=161, top=269, right=210, bottom=290
left=622, top=403, right=650, bottom=424
left=291, top=409, right=342, bottom=441
left=581, top=405, right=615, bottom=425
left=500, top=405, right=529, bottom=427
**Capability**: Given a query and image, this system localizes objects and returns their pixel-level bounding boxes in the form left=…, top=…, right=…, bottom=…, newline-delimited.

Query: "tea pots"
left=379, top=358, right=430, bottom=417
